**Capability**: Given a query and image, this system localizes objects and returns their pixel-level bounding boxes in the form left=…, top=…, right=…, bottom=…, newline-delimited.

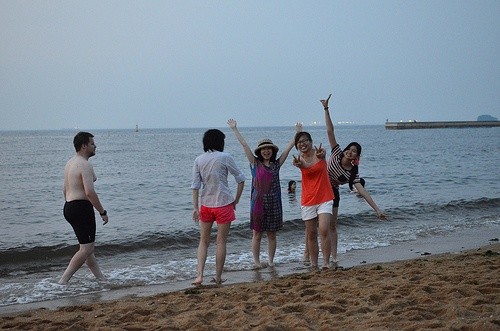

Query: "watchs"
left=100, top=210, right=107, bottom=216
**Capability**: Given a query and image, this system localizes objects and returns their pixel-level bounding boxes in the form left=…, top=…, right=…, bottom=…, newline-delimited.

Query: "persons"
left=227, top=118, right=302, bottom=268
left=303, top=94, right=391, bottom=264
left=287, top=181, right=296, bottom=193
left=57, top=131, right=108, bottom=284
left=291, top=132, right=336, bottom=269
left=191, top=129, right=246, bottom=284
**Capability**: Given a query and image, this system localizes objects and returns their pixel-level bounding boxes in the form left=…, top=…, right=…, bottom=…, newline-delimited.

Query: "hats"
left=254, top=139, right=279, bottom=156
